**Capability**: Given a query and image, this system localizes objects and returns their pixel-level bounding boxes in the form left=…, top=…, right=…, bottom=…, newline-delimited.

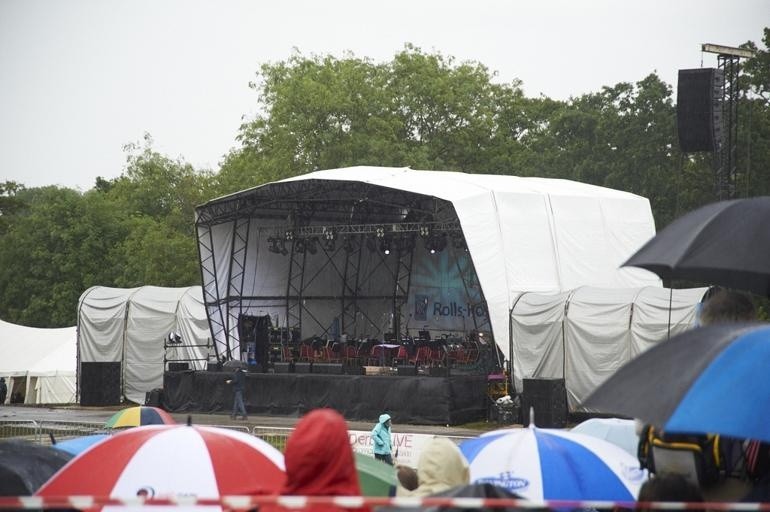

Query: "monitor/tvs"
left=419, top=331, right=431, bottom=340
left=383, top=333, right=395, bottom=343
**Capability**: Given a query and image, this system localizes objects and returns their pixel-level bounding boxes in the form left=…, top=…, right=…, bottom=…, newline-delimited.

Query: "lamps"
left=264, top=233, right=446, bottom=255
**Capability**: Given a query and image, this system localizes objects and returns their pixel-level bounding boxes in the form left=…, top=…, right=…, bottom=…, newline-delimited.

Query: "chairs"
left=282, top=344, right=479, bottom=370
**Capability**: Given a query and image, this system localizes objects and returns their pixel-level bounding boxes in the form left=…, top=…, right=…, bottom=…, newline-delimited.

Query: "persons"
left=371, top=413, right=393, bottom=464
left=0, top=378, right=7, bottom=406
left=257, top=407, right=371, bottom=512
left=408, top=437, right=471, bottom=499
left=229, top=366, right=248, bottom=421
left=398, top=467, right=418, bottom=494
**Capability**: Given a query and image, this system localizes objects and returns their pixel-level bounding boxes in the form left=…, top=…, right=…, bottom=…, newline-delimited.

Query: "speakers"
left=208, top=360, right=224, bottom=370
left=523, top=377, right=567, bottom=428
left=80, top=361, right=121, bottom=407
left=677, top=68, right=724, bottom=153
left=274, top=362, right=344, bottom=375
left=170, top=363, right=189, bottom=370
left=398, top=365, right=417, bottom=375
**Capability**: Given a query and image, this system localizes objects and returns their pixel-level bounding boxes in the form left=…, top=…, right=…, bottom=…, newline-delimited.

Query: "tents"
left=0, top=320, right=79, bottom=404
left=75, top=285, right=218, bottom=407
left=506, top=284, right=711, bottom=414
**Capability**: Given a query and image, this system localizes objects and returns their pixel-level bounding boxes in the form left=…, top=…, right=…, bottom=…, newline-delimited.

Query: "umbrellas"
left=30, top=414, right=287, bottom=511
left=51, top=434, right=112, bottom=458
left=618, top=194, right=769, bottom=296
left=352, top=450, right=394, bottom=497
left=102, top=405, right=177, bottom=429
left=0, top=439, right=75, bottom=511
left=222, top=360, right=249, bottom=371
